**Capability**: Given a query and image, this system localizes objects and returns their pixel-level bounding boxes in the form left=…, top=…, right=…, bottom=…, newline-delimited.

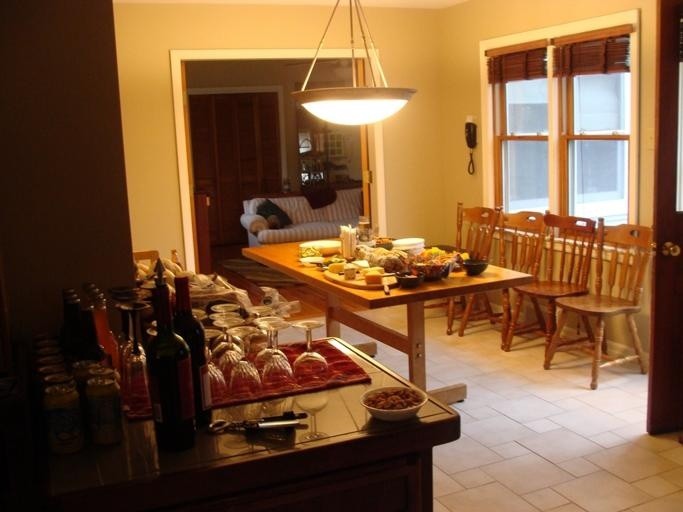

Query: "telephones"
left=465, top=122, right=477, bottom=148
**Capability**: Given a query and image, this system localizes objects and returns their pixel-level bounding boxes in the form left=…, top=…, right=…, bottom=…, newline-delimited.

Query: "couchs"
left=239, top=187, right=366, bottom=250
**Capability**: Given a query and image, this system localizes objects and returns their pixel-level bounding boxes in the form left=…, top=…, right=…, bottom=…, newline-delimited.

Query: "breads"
left=328, top=263, right=344, bottom=273
left=345, top=269, right=356, bottom=280
left=366, top=271, right=383, bottom=284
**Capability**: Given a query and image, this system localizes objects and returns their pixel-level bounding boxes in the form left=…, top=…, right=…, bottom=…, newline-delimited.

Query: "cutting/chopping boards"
left=324, top=269, right=398, bottom=289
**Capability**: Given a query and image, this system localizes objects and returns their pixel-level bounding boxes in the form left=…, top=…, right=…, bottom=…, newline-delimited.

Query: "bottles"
left=61, top=280, right=119, bottom=372
left=143, top=273, right=214, bottom=452
left=170, top=250, right=183, bottom=271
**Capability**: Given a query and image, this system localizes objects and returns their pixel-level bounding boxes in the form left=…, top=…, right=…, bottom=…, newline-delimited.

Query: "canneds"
left=36, top=335, right=125, bottom=452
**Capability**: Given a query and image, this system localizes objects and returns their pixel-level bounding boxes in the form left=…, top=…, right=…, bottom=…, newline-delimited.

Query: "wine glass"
left=116, top=296, right=328, bottom=404
left=216, top=392, right=334, bottom=457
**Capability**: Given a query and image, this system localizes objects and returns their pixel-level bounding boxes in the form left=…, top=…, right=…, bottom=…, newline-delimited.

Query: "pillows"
left=256, top=199, right=293, bottom=229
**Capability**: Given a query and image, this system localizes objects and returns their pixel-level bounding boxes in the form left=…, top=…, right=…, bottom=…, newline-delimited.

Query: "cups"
left=356, top=216, right=380, bottom=244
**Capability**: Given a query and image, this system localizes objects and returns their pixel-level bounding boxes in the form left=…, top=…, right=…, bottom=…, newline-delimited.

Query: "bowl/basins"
left=360, top=385, right=433, bottom=421
left=375, top=236, right=489, bottom=288
left=299, top=240, right=345, bottom=267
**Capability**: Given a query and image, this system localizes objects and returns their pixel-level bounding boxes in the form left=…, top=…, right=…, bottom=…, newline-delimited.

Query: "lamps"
left=287, top=0, right=419, bottom=130
